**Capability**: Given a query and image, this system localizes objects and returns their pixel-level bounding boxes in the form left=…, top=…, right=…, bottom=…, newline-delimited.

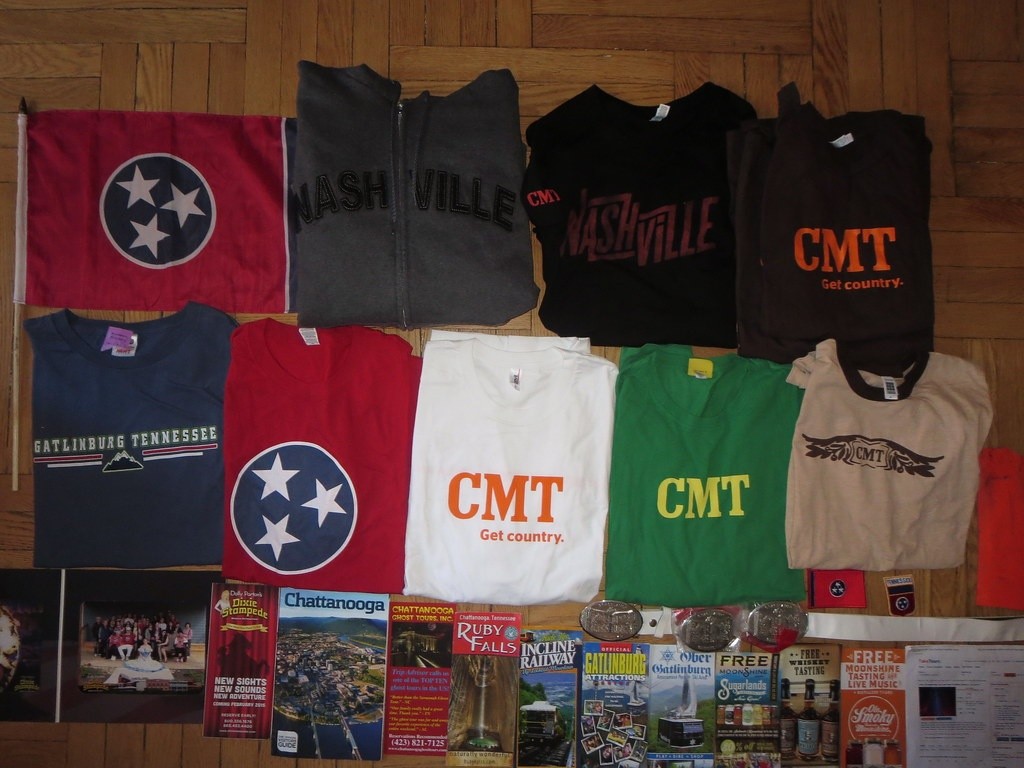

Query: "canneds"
left=717, top=704, right=778, bottom=725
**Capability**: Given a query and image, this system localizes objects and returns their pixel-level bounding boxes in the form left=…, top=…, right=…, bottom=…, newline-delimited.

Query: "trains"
left=520, top=699, right=568, bottom=742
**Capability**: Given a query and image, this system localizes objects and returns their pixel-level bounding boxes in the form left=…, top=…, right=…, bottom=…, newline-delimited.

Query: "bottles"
left=884, top=739, right=902, bottom=768
left=863, top=740, right=884, bottom=768
left=797, top=679, right=820, bottom=761
left=777, top=678, right=797, bottom=760
left=717, top=704, right=779, bottom=726
left=847, top=742, right=863, bottom=768
left=820, top=680, right=840, bottom=761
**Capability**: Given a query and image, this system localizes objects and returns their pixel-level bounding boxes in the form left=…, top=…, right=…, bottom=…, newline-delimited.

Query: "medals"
left=746, top=600, right=808, bottom=646
left=578, top=599, right=645, bottom=642
left=678, top=608, right=737, bottom=651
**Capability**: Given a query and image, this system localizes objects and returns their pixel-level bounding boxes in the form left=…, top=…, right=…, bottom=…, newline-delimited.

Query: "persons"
left=582, top=701, right=646, bottom=764
left=94, top=614, right=192, bottom=664
